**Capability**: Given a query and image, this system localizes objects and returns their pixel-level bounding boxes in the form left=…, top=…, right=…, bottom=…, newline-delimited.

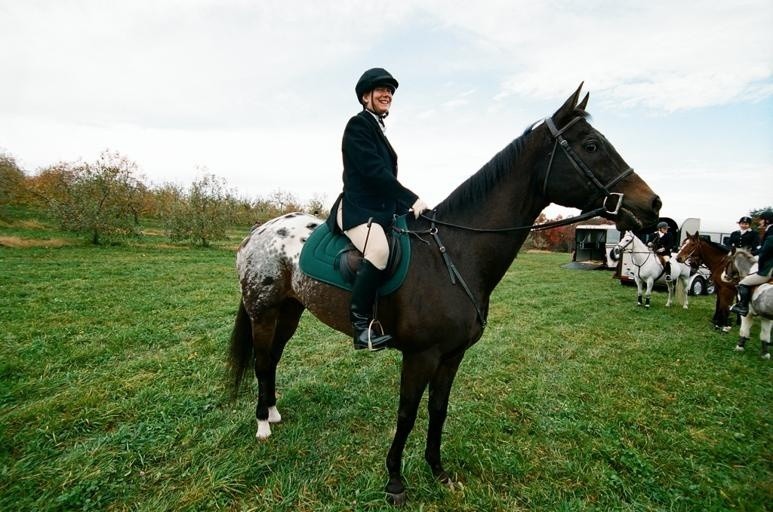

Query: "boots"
left=731, top=284, right=750, bottom=317
left=666, top=261, right=672, bottom=280
left=349, top=259, right=393, bottom=350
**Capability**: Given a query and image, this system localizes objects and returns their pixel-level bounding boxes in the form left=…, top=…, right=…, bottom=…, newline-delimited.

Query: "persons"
left=324, top=67, right=428, bottom=350
left=728, top=211, right=773, bottom=317
left=645, top=221, right=673, bottom=282
left=723, top=216, right=762, bottom=283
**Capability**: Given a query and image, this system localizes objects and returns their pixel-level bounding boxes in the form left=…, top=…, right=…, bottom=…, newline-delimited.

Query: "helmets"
left=737, top=216, right=752, bottom=224
left=759, top=211, right=773, bottom=221
left=657, top=221, right=670, bottom=228
left=355, top=68, right=399, bottom=101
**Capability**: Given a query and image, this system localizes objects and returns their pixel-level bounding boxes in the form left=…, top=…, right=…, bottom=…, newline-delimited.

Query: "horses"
left=674, top=230, right=773, bottom=360
left=614, top=230, right=692, bottom=310
left=219, top=80, right=663, bottom=505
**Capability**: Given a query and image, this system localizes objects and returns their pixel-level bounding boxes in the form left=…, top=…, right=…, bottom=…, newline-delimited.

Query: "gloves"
left=412, top=199, right=427, bottom=218
